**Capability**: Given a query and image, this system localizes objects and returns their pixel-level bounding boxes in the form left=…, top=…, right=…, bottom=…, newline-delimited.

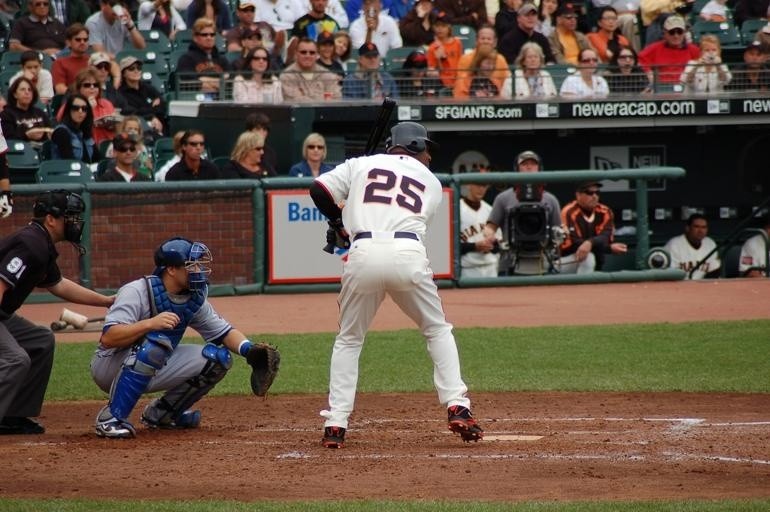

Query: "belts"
left=354, top=232, right=418, bottom=242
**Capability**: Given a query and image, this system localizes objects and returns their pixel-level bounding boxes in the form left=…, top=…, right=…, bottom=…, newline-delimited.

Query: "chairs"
left=0, top=0, right=209, bottom=182
left=172, top=3, right=770, bottom=100
left=600, top=204, right=770, bottom=272
left=212, top=136, right=386, bottom=180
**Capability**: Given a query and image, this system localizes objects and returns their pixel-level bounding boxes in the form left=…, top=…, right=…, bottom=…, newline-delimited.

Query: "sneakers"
left=0, top=415, right=46, bottom=435
left=139, top=408, right=202, bottom=430
left=321, top=426, right=347, bottom=449
left=95, top=419, right=139, bottom=438
left=446, top=404, right=485, bottom=443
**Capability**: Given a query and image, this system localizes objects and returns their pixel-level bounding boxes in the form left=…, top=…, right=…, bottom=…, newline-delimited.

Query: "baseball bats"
left=324, top=96, right=395, bottom=255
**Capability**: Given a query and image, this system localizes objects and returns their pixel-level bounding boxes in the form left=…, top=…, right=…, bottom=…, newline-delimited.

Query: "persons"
left=0, top=190, right=118, bottom=435
left=89, top=238, right=280, bottom=439
left=309, top=121, right=484, bottom=450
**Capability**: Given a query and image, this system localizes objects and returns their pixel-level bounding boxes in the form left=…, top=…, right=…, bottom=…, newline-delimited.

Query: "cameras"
left=707, top=54, right=716, bottom=60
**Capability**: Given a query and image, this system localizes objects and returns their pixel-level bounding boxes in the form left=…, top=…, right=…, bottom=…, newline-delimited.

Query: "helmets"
left=31, top=187, right=87, bottom=245
left=384, top=120, right=439, bottom=154
left=152, top=236, right=214, bottom=300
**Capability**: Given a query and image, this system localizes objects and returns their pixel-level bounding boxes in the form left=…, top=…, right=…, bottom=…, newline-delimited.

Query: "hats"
left=744, top=38, right=770, bottom=56
left=555, top=2, right=580, bottom=19
left=574, top=178, right=605, bottom=192
left=358, top=41, right=381, bottom=57
left=517, top=4, right=538, bottom=17
left=113, top=130, right=139, bottom=149
left=515, top=149, right=541, bottom=167
left=429, top=11, right=453, bottom=26
left=239, top=2, right=258, bottom=12
left=662, top=15, right=687, bottom=34
left=406, top=50, right=429, bottom=70
left=317, top=29, right=335, bottom=46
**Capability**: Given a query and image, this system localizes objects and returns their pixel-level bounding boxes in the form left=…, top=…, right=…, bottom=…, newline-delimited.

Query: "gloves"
left=324, top=220, right=352, bottom=251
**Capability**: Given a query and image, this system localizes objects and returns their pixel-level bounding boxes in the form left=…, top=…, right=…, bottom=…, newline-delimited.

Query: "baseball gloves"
left=247, top=343, right=281, bottom=397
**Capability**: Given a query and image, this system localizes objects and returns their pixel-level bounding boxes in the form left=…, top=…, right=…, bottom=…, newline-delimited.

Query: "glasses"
left=65, top=50, right=142, bottom=115
left=108, top=0, right=125, bottom=7
left=618, top=54, right=635, bottom=60
left=298, top=49, right=317, bottom=56
left=583, top=189, right=602, bottom=196
left=32, top=1, right=49, bottom=8
left=197, top=31, right=217, bottom=38
left=306, top=144, right=326, bottom=150
left=117, top=145, right=137, bottom=153
left=183, top=140, right=207, bottom=148
left=74, top=36, right=89, bottom=43
left=668, top=28, right=683, bottom=36
left=239, top=22, right=271, bottom=62
left=252, top=145, right=265, bottom=151
left=579, top=57, right=598, bottom=64
